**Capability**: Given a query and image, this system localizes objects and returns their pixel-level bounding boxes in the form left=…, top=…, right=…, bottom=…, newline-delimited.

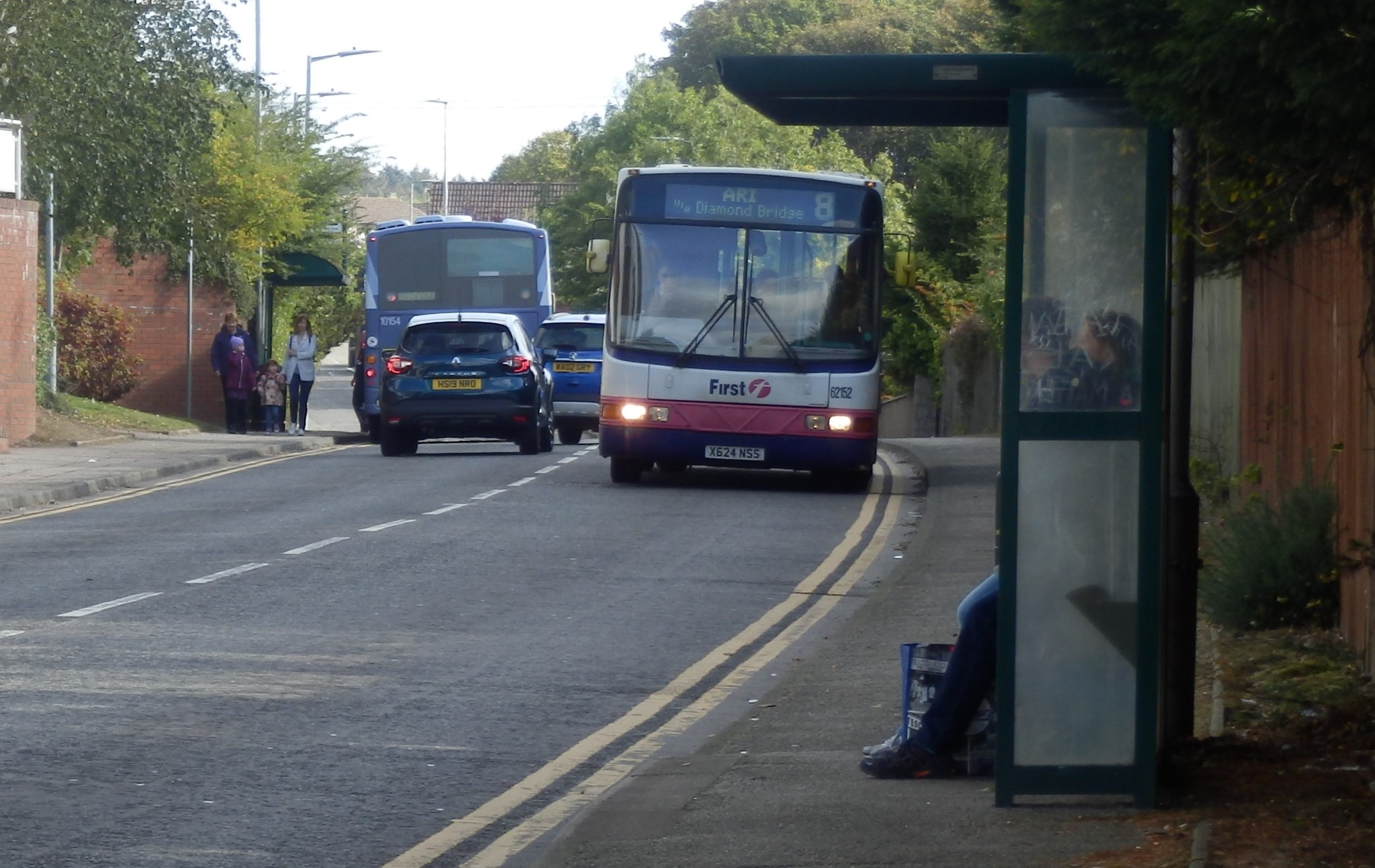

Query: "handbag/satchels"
left=899, top=641, right=994, bottom=778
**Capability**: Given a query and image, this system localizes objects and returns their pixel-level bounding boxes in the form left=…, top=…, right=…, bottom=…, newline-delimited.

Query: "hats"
left=230, top=336, right=244, bottom=349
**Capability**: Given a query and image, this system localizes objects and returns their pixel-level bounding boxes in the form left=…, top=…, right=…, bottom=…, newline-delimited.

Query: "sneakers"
left=860, top=739, right=955, bottom=779
left=863, top=735, right=903, bottom=755
left=298, top=429, right=305, bottom=436
left=288, top=422, right=296, bottom=435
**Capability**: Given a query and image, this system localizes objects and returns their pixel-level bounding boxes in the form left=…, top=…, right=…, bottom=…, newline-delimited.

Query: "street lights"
left=293, top=49, right=382, bottom=154
left=410, top=180, right=431, bottom=223
left=428, top=100, right=448, bottom=215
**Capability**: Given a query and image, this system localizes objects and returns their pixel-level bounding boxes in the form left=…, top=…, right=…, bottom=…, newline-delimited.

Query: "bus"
left=586, top=164, right=916, bottom=495
left=364, top=215, right=555, bottom=444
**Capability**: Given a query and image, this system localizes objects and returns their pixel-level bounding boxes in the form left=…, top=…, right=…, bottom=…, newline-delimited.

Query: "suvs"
left=531, top=312, right=607, bottom=444
left=380, top=313, right=558, bottom=456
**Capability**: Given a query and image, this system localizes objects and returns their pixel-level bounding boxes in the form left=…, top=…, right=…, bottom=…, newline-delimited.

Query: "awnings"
left=262, top=251, right=348, bottom=287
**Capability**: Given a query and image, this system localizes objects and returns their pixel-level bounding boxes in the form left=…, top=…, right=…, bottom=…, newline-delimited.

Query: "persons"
left=859, top=301, right=1140, bottom=780
left=352, top=330, right=367, bottom=432
left=654, top=268, right=699, bottom=313
left=759, top=268, right=864, bottom=342
left=211, top=311, right=317, bottom=437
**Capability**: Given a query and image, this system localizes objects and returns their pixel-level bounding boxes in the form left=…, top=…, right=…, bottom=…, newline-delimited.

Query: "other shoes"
left=239, top=428, right=246, bottom=434
left=274, top=424, right=280, bottom=433
left=227, top=425, right=236, bottom=434
left=265, top=431, right=270, bottom=435
